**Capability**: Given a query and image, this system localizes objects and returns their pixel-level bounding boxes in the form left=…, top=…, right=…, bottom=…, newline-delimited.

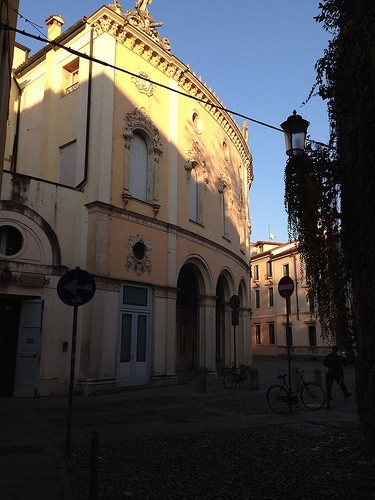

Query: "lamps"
left=281, top=110, right=339, bottom=167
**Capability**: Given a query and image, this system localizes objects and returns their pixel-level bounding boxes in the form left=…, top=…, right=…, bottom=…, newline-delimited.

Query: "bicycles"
left=265, top=368, right=327, bottom=414
left=222, top=362, right=251, bottom=390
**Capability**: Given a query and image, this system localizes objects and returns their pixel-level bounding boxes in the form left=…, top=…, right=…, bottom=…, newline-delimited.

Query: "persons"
left=323, top=345, right=353, bottom=409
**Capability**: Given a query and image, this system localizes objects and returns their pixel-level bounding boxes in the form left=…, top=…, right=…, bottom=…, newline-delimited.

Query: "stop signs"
left=278, top=276, right=295, bottom=298
